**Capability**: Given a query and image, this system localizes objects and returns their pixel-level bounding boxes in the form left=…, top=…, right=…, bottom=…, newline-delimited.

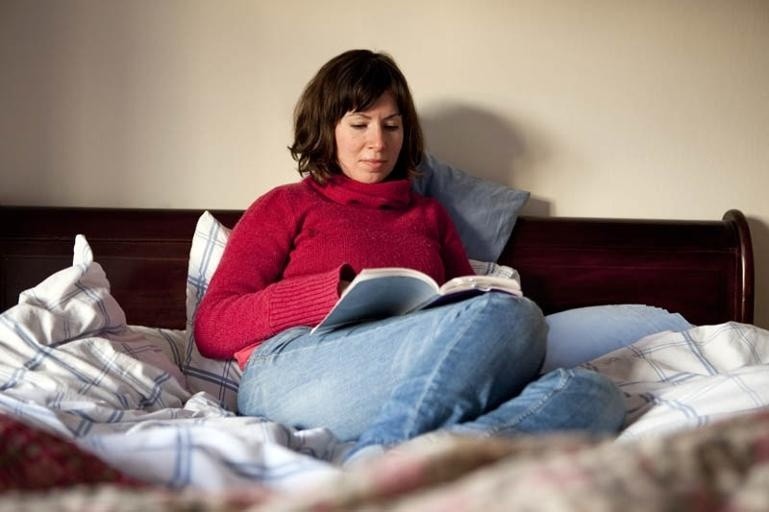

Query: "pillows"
left=415, top=150, right=533, bottom=262
left=184, top=210, right=522, bottom=414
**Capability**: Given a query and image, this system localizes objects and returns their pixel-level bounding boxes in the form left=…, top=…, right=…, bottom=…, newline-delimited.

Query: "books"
left=306, top=265, right=527, bottom=343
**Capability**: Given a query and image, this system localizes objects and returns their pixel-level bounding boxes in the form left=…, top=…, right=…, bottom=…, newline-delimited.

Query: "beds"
left=1, top=203, right=766, bottom=512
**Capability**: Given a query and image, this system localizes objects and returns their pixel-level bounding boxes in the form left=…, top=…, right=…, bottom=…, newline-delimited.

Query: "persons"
left=193, top=45, right=628, bottom=460
